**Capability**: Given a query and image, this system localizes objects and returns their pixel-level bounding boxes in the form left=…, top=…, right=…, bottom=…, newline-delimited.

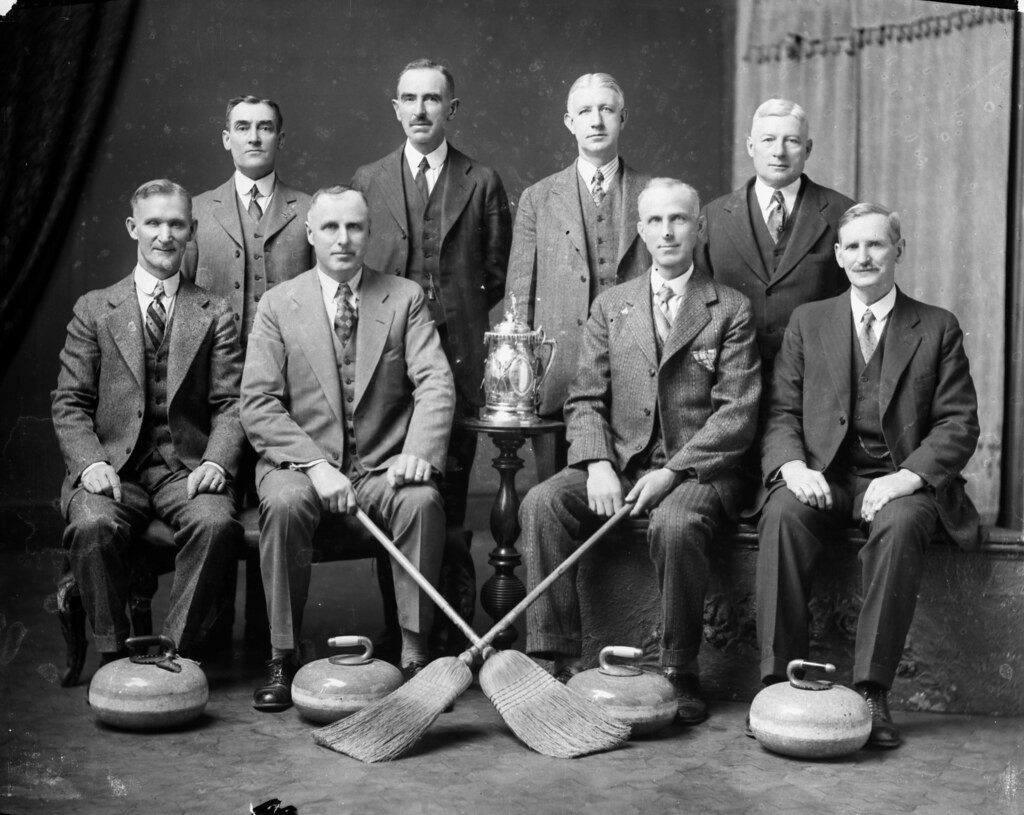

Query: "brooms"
left=312, top=502, right=637, bottom=762
left=351, top=505, right=635, bottom=761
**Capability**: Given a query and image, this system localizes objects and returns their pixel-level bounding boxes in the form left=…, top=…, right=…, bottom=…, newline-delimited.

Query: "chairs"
left=58, top=447, right=464, bottom=692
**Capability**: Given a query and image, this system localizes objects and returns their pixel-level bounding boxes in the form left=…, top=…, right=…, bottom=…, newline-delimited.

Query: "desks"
left=459, top=416, right=566, bottom=689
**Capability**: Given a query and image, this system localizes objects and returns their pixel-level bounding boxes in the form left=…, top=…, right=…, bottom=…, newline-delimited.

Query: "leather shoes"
left=666, top=671, right=708, bottom=724
left=551, top=665, right=578, bottom=685
left=854, top=681, right=901, bottom=748
left=403, top=662, right=453, bottom=715
left=252, top=655, right=295, bottom=710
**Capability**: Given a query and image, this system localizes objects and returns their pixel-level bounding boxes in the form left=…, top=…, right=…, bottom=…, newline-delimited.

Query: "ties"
left=858, top=312, right=873, bottom=364
left=335, top=283, right=354, bottom=343
left=657, top=286, right=675, bottom=314
left=592, top=170, right=604, bottom=204
left=143, top=284, right=166, bottom=348
left=770, top=191, right=785, bottom=214
left=414, top=157, right=428, bottom=201
left=247, top=184, right=263, bottom=223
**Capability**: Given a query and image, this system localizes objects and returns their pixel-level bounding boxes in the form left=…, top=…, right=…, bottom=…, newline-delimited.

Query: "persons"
left=239, top=186, right=458, bottom=714
left=690, top=98, right=858, bottom=379
left=49, top=177, right=245, bottom=693
left=744, top=202, right=980, bottom=748
left=180, top=95, right=315, bottom=355
left=504, top=72, right=660, bottom=480
left=345, top=59, right=513, bottom=665
left=517, top=178, right=760, bottom=723
